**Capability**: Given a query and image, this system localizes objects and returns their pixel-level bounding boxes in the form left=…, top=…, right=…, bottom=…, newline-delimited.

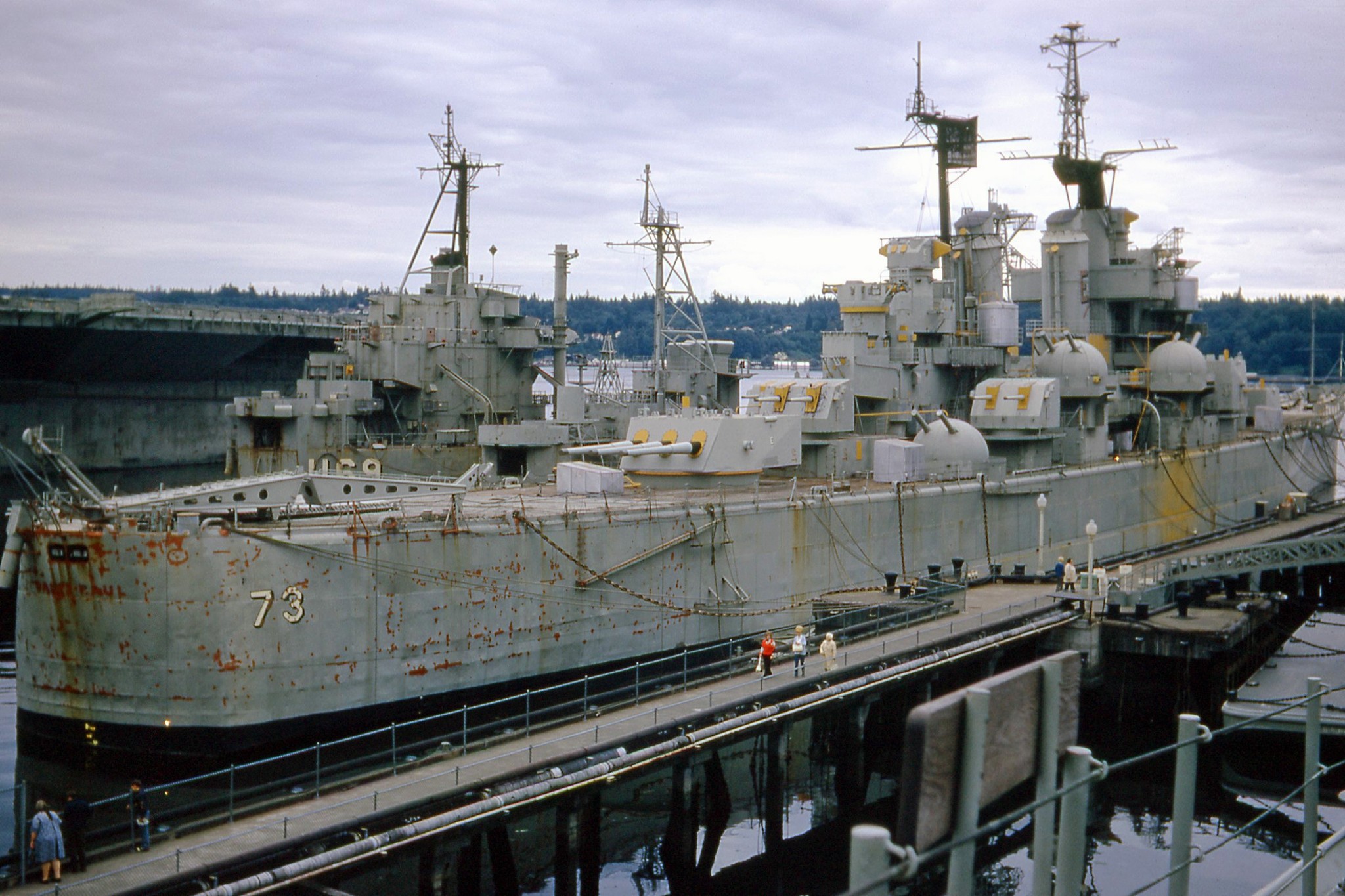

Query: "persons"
left=30, top=800, right=64, bottom=885
left=759, top=631, right=775, bottom=677
left=126, top=779, right=151, bottom=853
left=1055, top=556, right=1065, bottom=592
left=819, top=632, right=837, bottom=672
left=792, top=625, right=807, bottom=678
left=1063, top=558, right=1077, bottom=593
left=67, top=790, right=91, bottom=874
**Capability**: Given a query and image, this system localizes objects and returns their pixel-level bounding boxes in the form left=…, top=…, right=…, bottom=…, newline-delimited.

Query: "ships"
left=1, top=19, right=1345, bottom=732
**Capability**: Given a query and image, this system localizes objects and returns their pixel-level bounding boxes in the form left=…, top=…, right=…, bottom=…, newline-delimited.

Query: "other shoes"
left=68, top=867, right=80, bottom=873
left=136, top=847, right=149, bottom=852
left=34, top=877, right=48, bottom=884
left=50, top=876, right=61, bottom=882
left=79, top=867, right=86, bottom=872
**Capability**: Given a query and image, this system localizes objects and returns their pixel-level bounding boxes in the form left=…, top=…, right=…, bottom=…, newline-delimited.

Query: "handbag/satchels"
left=755, top=657, right=762, bottom=672
left=792, top=643, right=803, bottom=652
left=831, top=660, right=838, bottom=670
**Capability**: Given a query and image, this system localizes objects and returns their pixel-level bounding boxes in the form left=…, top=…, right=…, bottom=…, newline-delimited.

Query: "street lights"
left=1085, top=519, right=1098, bottom=590
left=1036, top=492, right=1047, bottom=576
